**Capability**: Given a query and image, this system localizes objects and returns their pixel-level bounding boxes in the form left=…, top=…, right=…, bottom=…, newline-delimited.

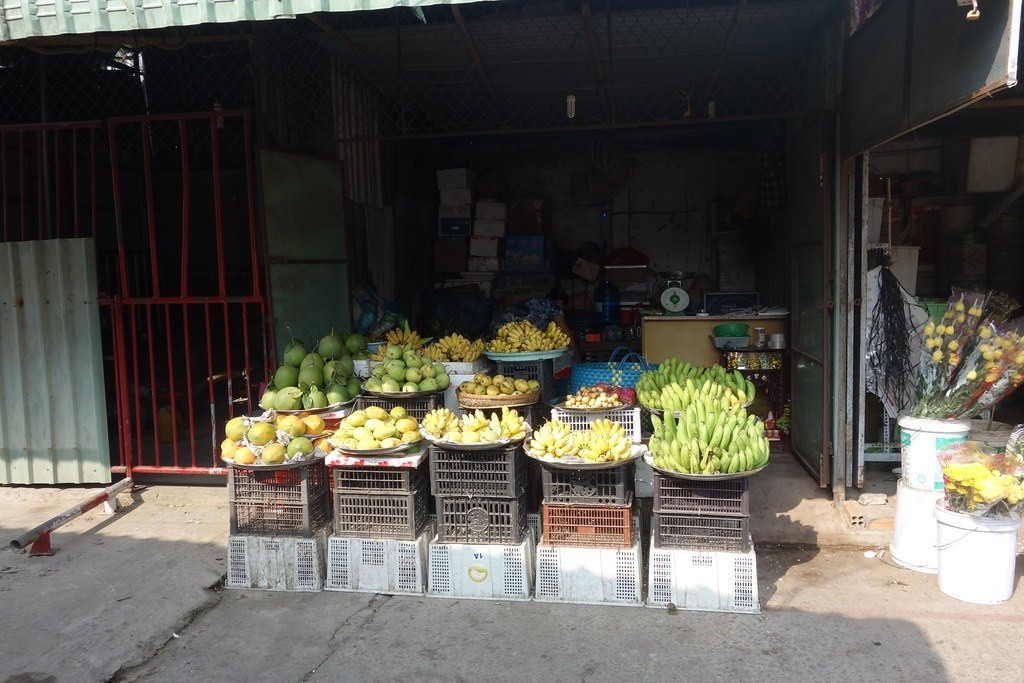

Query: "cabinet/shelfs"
left=717, top=346, right=791, bottom=454
left=604, top=247, right=650, bottom=307
left=639, top=313, right=787, bottom=367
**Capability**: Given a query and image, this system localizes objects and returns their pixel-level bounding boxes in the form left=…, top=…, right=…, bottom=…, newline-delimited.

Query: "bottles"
left=593, top=281, right=621, bottom=326
left=570, top=327, right=591, bottom=342
left=753, top=326, right=765, bottom=347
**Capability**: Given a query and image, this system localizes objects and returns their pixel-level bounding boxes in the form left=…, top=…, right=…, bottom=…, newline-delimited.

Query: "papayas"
left=220, top=415, right=331, bottom=464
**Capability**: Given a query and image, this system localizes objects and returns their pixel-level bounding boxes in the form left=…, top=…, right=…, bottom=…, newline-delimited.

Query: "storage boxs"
left=504, top=235, right=545, bottom=271
left=226, top=342, right=760, bottom=614
left=434, top=239, right=466, bottom=273
left=438, top=203, right=472, bottom=219
left=475, top=201, right=507, bottom=220
left=439, top=219, right=471, bottom=236
left=941, top=134, right=1024, bottom=192
left=467, top=257, right=500, bottom=272
left=436, top=168, right=467, bottom=191
left=509, top=193, right=552, bottom=236
left=474, top=220, right=506, bottom=238
left=469, top=235, right=499, bottom=257
left=440, top=187, right=472, bottom=203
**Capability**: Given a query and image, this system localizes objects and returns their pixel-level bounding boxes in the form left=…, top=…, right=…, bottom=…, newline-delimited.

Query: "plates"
left=639, top=401, right=754, bottom=418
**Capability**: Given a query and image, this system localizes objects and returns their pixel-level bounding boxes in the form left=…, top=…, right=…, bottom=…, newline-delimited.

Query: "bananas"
left=487, top=319, right=570, bottom=353
left=370, top=327, right=485, bottom=362
left=648, top=393, right=770, bottom=475
left=636, top=357, right=757, bottom=412
left=421, top=406, right=527, bottom=442
left=528, top=418, right=631, bottom=463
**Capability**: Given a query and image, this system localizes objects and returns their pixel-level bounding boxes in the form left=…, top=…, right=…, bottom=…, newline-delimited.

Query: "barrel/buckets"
left=888, top=408, right=1024, bottom=606
left=620, top=308, right=637, bottom=329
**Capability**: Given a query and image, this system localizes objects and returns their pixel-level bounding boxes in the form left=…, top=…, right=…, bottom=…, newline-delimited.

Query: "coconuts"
left=261, top=332, right=367, bottom=409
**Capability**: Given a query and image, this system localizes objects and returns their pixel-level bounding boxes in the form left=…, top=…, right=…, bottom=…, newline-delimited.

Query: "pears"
left=364, top=345, right=450, bottom=393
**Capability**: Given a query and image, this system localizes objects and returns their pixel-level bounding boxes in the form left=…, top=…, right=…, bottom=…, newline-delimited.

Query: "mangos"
left=459, top=374, right=541, bottom=397
left=332, top=406, right=422, bottom=449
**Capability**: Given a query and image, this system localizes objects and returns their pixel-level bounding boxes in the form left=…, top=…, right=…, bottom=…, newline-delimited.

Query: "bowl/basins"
left=366, top=341, right=387, bottom=354
left=713, top=323, right=750, bottom=337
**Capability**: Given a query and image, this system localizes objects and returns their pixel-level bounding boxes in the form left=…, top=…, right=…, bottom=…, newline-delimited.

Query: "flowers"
left=910, top=287, right=1024, bottom=420
left=937, top=427, right=1024, bottom=518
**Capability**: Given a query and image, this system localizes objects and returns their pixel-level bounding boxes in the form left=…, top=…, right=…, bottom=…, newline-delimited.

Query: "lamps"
left=566, top=94, right=576, bottom=118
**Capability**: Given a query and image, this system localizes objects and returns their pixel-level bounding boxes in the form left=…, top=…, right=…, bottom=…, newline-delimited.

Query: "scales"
left=660, top=270, right=695, bottom=315
